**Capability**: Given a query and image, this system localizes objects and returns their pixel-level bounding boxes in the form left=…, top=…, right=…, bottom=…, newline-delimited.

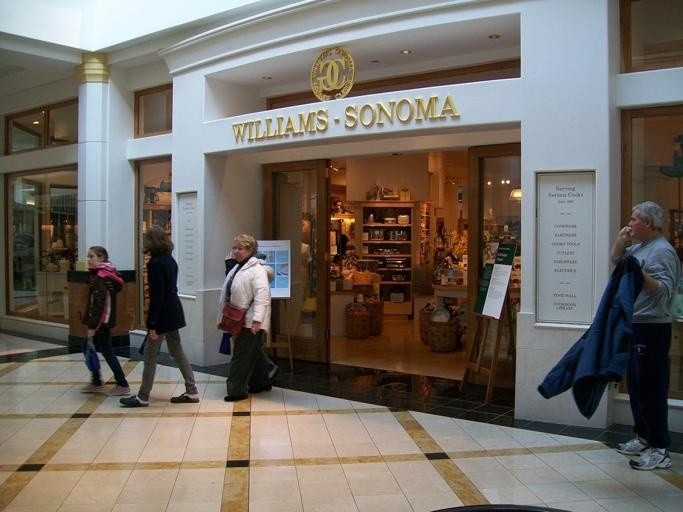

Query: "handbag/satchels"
left=217, top=303, right=247, bottom=338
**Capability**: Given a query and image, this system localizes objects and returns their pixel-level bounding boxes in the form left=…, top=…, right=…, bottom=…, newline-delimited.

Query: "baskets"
left=344, top=303, right=371, bottom=340
left=417, top=305, right=434, bottom=345
left=355, top=296, right=384, bottom=337
left=429, top=319, right=455, bottom=352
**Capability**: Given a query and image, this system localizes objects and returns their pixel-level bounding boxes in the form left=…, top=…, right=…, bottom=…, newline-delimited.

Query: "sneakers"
left=628, top=449, right=672, bottom=470
left=169, top=391, right=201, bottom=403
left=79, top=384, right=130, bottom=396
left=615, top=438, right=648, bottom=455
left=222, top=361, right=281, bottom=401
left=118, top=394, right=149, bottom=407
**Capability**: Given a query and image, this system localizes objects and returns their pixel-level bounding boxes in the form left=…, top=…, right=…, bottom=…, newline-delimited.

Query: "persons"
left=224, top=244, right=273, bottom=394
left=219, top=234, right=295, bottom=401
left=119, top=227, right=198, bottom=406
left=78, top=244, right=132, bottom=396
left=609, top=198, right=680, bottom=469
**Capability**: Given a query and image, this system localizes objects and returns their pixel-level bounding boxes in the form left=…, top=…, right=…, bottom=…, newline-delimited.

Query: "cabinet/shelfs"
left=143, top=204, right=171, bottom=329
left=35, top=269, right=69, bottom=321
left=329, top=200, right=521, bottom=337
